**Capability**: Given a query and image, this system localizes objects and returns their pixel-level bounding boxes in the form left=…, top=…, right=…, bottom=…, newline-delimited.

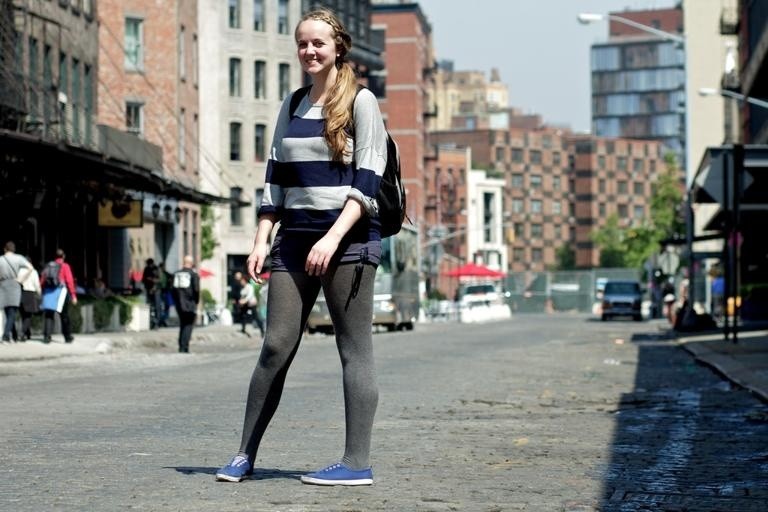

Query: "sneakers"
left=2, top=334, right=31, bottom=342
left=215, top=455, right=255, bottom=483
left=44, top=336, right=51, bottom=342
left=301, top=462, right=373, bottom=486
left=66, top=336, right=73, bottom=342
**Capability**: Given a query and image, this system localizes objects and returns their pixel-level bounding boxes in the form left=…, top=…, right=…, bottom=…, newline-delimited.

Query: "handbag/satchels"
left=20, top=290, right=40, bottom=314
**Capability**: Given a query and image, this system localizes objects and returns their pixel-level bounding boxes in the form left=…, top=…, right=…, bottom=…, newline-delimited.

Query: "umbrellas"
left=442, top=263, right=505, bottom=277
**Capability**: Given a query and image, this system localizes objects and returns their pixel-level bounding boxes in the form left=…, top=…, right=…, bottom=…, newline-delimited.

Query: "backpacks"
left=45, top=260, right=61, bottom=289
left=286, top=82, right=406, bottom=237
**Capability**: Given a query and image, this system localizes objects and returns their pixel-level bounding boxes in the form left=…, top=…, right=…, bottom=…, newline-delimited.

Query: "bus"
left=306, top=222, right=421, bottom=335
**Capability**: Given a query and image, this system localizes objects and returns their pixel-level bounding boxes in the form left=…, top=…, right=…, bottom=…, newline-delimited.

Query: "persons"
left=18, top=257, right=42, bottom=341
left=155, top=262, right=176, bottom=323
left=229, top=270, right=244, bottom=325
left=1, top=242, right=33, bottom=345
left=658, top=263, right=726, bottom=326
left=165, top=254, right=201, bottom=354
left=238, top=276, right=267, bottom=341
left=142, top=259, right=164, bottom=331
left=214, top=10, right=390, bottom=486
left=38, top=249, right=79, bottom=344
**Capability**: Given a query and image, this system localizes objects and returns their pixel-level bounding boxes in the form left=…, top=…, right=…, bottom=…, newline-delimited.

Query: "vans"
left=597, top=281, right=647, bottom=321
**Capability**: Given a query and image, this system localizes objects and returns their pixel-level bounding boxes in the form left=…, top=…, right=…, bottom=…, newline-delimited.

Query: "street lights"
left=700, top=88, right=768, bottom=118
left=573, top=12, right=696, bottom=334
